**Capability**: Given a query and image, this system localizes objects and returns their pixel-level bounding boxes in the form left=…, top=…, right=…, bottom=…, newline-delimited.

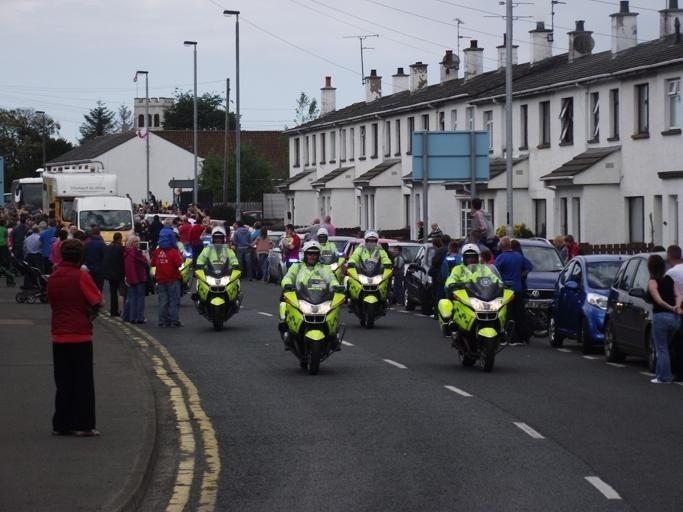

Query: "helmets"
left=316, top=228, right=328, bottom=242
left=364, top=231, right=378, bottom=244
left=212, top=226, right=227, bottom=242
left=299, top=240, right=322, bottom=261
left=462, top=243, right=481, bottom=264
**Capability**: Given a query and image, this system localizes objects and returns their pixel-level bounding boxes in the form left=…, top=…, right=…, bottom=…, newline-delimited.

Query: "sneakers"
left=158, top=322, right=171, bottom=328
left=52, top=429, right=85, bottom=436
left=500, top=340, right=524, bottom=346
left=171, top=322, right=184, bottom=327
left=86, top=428, right=100, bottom=434
left=650, top=378, right=672, bottom=384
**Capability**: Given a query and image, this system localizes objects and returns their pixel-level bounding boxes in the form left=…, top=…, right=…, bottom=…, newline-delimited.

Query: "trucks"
left=42, top=164, right=118, bottom=233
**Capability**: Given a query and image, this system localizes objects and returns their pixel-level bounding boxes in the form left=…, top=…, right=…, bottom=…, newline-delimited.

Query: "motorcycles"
left=307, top=252, right=346, bottom=295
left=188, top=253, right=246, bottom=329
left=344, top=257, right=396, bottom=323
left=276, top=267, right=346, bottom=373
left=437, top=277, right=517, bottom=367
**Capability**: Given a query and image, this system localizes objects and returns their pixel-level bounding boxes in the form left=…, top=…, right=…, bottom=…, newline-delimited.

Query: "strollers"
left=0, top=263, right=16, bottom=288
left=12, top=260, right=50, bottom=303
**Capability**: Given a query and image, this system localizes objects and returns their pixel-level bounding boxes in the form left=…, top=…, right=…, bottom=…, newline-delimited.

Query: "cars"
left=268, top=231, right=308, bottom=280
left=547, top=254, right=632, bottom=356
left=144, top=214, right=196, bottom=230
left=329, top=237, right=426, bottom=306
left=402, top=244, right=460, bottom=316
left=602, top=251, right=671, bottom=371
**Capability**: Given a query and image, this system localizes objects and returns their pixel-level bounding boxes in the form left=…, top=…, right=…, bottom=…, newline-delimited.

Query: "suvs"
left=507, top=237, right=565, bottom=339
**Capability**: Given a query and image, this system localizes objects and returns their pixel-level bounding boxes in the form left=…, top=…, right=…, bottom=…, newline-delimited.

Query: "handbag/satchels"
left=120, top=280, right=126, bottom=296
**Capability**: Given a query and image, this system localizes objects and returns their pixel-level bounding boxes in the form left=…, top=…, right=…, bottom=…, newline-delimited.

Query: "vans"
left=73, top=196, right=136, bottom=248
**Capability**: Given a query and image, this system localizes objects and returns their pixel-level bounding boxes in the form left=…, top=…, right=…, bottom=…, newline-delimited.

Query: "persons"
left=228, top=214, right=338, bottom=287
left=344, top=231, right=392, bottom=314
left=5, top=194, right=212, bottom=327
left=380, top=199, right=578, bottom=342
left=277, top=240, right=342, bottom=350
left=44, top=238, right=108, bottom=439
left=192, top=226, right=242, bottom=316
left=646, top=242, right=683, bottom=386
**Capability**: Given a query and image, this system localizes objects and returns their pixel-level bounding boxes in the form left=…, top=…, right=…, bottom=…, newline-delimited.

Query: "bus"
left=11, top=177, right=42, bottom=213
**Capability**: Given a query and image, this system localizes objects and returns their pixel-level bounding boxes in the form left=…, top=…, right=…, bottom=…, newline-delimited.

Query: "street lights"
left=221, top=9, right=241, bottom=221
left=134, top=69, right=150, bottom=202
left=34, top=110, right=45, bottom=165
left=181, top=39, right=197, bottom=207
